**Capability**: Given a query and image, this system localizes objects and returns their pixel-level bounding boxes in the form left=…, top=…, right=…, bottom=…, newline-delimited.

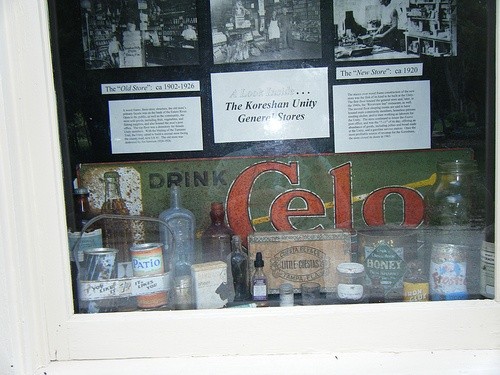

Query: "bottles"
left=225, top=234, right=252, bottom=302
left=423, top=155, right=494, bottom=300
left=251, top=252, right=270, bottom=307
left=158, top=184, right=197, bottom=272
left=173, top=275, right=194, bottom=310
left=201, top=200, right=234, bottom=263
left=69, top=186, right=101, bottom=232
left=278, top=282, right=295, bottom=307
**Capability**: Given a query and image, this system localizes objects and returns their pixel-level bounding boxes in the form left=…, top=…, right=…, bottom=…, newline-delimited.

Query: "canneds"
left=336, top=263, right=364, bottom=301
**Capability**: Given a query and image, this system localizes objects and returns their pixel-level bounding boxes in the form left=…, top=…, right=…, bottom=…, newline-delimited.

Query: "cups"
left=300, top=282, right=321, bottom=306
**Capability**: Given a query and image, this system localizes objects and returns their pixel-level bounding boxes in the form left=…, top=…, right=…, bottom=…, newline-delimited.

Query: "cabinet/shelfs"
left=401, top=0, right=453, bottom=56
left=91, top=11, right=199, bottom=58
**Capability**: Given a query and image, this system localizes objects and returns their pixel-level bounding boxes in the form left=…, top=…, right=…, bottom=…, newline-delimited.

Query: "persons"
left=107, top=35, right=123, bottom=66
left=181, top=25, right=195, bottom=39
left=265, top=7, right=294, bottom=51
left=372, top=0, right=400, bottom=51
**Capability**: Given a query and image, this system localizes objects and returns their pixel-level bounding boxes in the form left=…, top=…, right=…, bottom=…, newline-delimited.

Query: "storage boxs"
left=247, top=227, right=351, bottom=294
